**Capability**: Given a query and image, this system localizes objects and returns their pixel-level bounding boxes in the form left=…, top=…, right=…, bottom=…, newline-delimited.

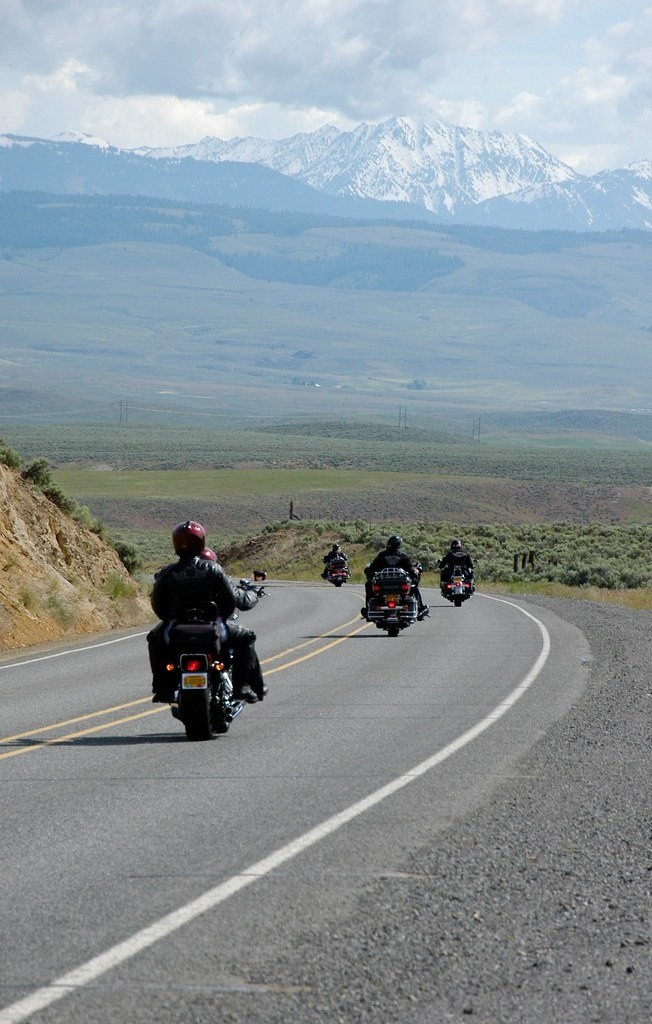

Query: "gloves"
left=248, top=585, right=260, bottom=595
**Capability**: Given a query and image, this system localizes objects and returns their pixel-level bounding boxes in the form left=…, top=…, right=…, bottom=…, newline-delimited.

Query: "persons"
left=357, top=535, right=430, bottom=622
left=144, top=519, right=259, bottom=706
left=177, top=546, right=270, bottom=702
left=433, top=538, right=476, bottom=593
left=320, top=542, right=350, bottom=580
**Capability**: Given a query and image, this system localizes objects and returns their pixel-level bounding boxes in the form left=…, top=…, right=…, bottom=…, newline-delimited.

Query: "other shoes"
left=361, top=608, right=370, bottom=622
left=321, top=573, right=326, bottom=579
left=417, top=605, right=430, bottom=622
left=257, top=685, right=269, bottom=697
left=152, top=690, right=178, bottom=703
left=233, top=685, right=257, bottom=699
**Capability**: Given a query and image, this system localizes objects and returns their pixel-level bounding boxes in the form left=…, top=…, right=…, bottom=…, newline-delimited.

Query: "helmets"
left=200, top=548, right=217, bottom=563
left=172, top=520, right=206, bottom=555
left=450, top=540, right=462, bottom=549
left=333, top=544, right=339, bottom=551
left=387, top=536, right=402, bottom=549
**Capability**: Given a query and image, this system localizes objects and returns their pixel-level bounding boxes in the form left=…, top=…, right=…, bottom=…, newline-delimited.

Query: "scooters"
left=437, top=556, right=477, bottom=606
left=363, top=559, right=425, bottom=638
left=148, top=566, right=271, bottom=741
left=322, top=552, right=350, bottom=588
